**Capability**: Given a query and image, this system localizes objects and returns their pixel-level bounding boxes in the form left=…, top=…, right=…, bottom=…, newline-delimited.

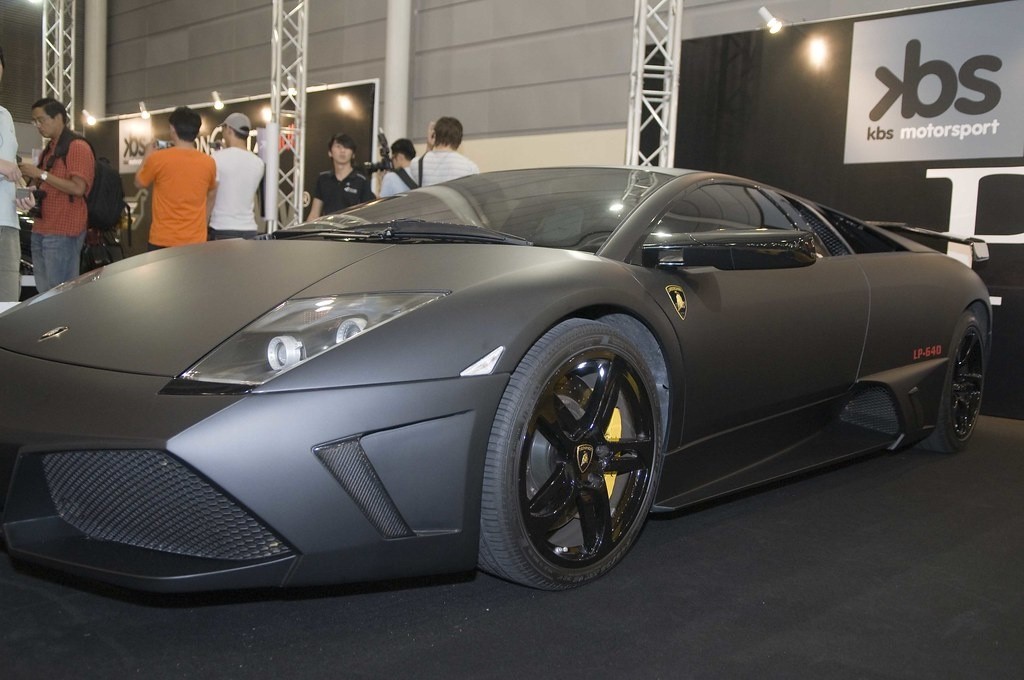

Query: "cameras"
left=156, top=140, right=171, bottom=148
left=364, top=127, right=394, bottom=176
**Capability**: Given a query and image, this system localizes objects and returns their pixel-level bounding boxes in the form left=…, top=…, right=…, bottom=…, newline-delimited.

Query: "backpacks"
left=43, top=128, right=124, bottom=231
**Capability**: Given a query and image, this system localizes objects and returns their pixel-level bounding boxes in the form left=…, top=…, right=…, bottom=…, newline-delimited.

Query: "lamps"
left=758, top=6, right=794, bottom=34
left=139, top=101, right=179, bottom=119
left=211, top=90, right=250, bottom=110
left=81, top=109, right=123, bottom=125
left=287, top=76, right=329, bottom=95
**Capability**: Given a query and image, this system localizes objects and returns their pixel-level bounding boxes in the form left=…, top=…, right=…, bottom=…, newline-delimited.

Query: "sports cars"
left=0, top=161, right=994, bottom=592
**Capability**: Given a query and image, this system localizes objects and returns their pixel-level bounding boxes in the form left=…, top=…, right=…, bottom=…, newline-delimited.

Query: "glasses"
left=31, top=118, right=51, bottom=126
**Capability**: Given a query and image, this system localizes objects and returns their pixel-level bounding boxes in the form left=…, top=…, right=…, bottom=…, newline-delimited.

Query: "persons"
left=17, top=99, right=95, bottom=293
left=307, top=133, right=369, bottom=221
left=409, top=117, right=481, bottom=188
left=380, top=139, right=416, bottom=198
left=207, top=112, right=265, bottom=242
left=0, top=51, right=21, bottom=302
left=134, top=107, right=216, bottom=251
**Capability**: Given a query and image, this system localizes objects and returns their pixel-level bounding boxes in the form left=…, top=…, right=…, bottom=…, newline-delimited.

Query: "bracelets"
left=41, top=172, right=48, bottom=180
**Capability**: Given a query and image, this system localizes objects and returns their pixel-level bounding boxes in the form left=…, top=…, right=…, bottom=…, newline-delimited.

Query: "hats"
left=217, top=113, right=250, bottom=138
